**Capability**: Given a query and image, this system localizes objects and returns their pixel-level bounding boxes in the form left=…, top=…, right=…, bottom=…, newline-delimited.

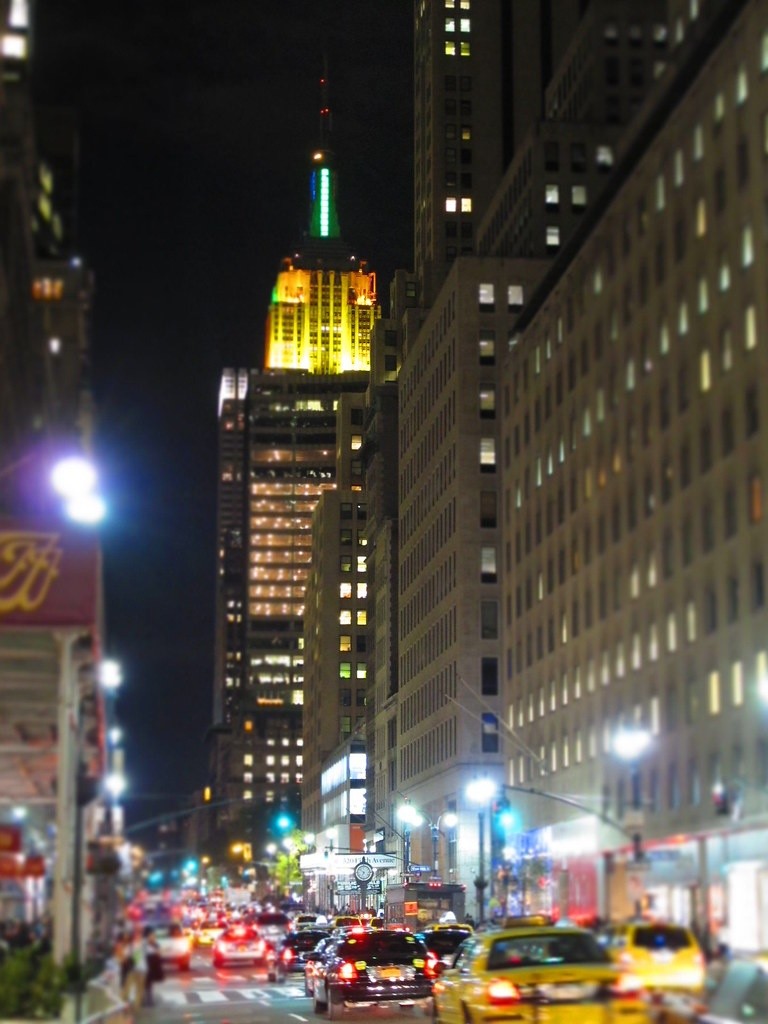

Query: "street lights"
left=461, top=775, right=645, bottom=919
left=396, top=802, right=458, bottom=877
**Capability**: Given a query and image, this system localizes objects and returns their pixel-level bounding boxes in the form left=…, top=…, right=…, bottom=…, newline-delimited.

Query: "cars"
left=598, top=917, right=709, bottom=1008
left=113, top=885, right=475, bottom=1020
left=428, top=912, right=651, bottom=1024
